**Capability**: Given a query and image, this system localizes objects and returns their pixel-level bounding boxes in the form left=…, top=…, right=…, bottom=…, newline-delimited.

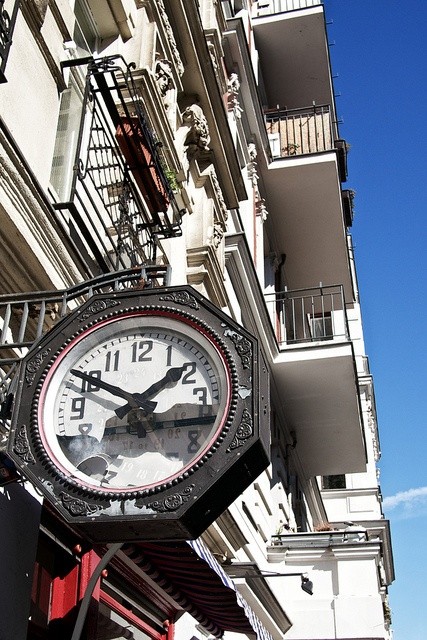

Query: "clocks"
left=4, top=284, right=272, bottom=544
